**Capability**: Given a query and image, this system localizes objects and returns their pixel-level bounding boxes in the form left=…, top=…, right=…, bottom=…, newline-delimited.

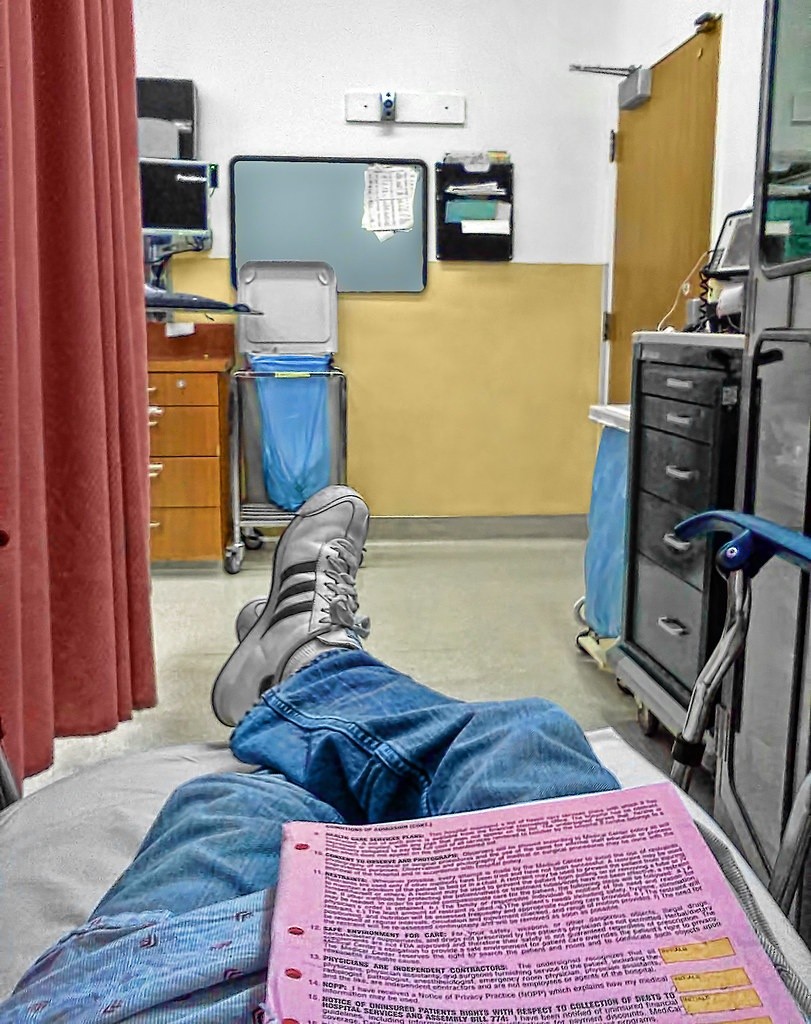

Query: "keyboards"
left=143, top=295, right=228, bottom=310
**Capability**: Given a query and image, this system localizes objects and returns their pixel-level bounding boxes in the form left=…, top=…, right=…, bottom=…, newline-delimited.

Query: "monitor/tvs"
left=705, top=207, right=754, bottom=277
left=139, top=158, right=210, bottom=234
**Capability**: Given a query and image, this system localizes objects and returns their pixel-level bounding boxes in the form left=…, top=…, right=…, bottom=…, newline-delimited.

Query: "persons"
left=0, top=484, right=619, bottom=1024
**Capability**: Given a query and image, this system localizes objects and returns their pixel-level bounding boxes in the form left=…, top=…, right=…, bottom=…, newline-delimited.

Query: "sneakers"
left=208, top=482, right=380, bottom=729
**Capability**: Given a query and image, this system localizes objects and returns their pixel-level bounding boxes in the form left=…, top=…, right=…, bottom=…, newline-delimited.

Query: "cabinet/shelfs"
left=144, top=351, right=233, bottom=569
left=626, top=364, right=727, bottom=707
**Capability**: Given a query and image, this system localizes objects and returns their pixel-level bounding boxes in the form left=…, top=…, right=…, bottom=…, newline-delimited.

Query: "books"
left=266, top=781, right=806, bottom=1023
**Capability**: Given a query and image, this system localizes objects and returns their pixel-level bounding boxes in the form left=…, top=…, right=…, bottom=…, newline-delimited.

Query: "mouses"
left=230, top=304, right=249, bottom=312
